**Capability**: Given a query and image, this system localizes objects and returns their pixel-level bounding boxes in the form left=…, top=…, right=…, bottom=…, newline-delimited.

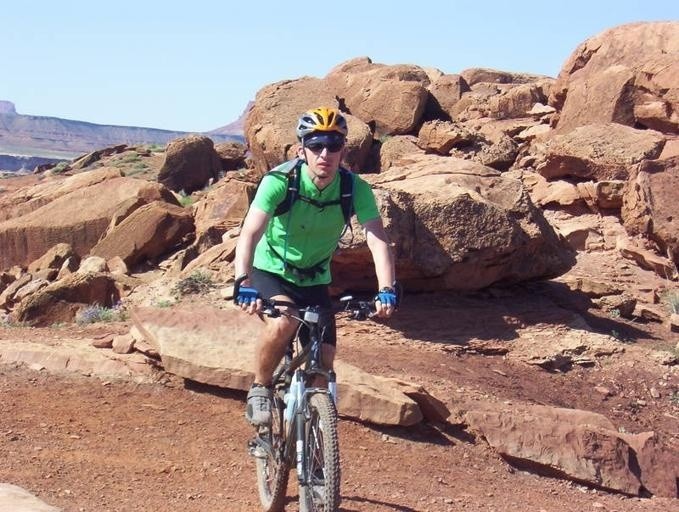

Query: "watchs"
left=378, top=286, right=394, bottom=293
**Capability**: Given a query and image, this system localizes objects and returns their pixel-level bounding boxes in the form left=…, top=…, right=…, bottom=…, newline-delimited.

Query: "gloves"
left=375, top=292, right=398, bottom=305
left=236, top=286, right=263, bottom=303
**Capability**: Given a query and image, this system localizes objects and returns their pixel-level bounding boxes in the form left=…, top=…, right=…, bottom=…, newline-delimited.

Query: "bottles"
left=284, top=367, right=305, bottom=425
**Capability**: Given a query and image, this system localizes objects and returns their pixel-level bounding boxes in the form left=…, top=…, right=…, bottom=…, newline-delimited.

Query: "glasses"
left=305, top=143, right=346, bottom=153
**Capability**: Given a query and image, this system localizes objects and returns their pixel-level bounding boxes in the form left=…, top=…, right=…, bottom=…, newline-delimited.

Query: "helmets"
left=295, top=106, right=348, bottom=143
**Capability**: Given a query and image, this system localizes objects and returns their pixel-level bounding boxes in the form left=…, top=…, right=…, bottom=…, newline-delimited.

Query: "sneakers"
left=245, top=381, right=275, bottom=427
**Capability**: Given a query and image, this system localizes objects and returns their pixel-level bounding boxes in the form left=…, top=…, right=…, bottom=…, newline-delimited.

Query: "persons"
left=231, top=104, right=403, bottom=488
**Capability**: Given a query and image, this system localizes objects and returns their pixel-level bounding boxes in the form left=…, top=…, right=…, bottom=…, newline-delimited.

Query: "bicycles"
left=222, top=292, right=400, bottom=512
